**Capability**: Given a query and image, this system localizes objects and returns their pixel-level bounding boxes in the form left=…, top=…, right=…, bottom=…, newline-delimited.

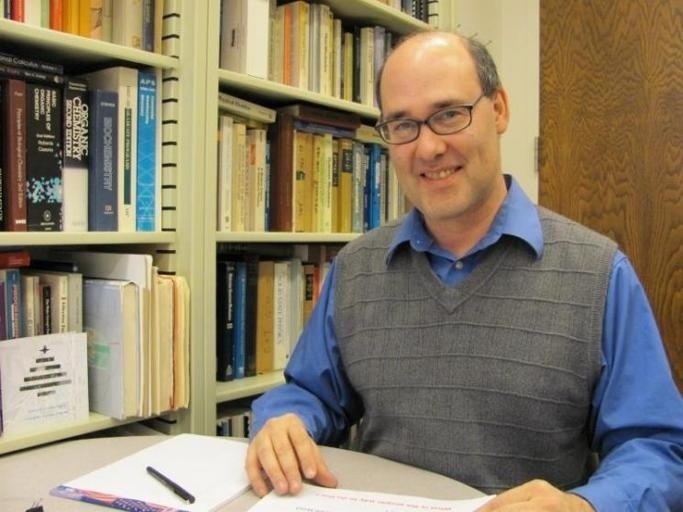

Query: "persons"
left=243, top=28, right=682, bottom=512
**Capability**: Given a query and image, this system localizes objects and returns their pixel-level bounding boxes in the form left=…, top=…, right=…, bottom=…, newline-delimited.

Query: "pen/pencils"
left=146, top=465, right=196, bottom=504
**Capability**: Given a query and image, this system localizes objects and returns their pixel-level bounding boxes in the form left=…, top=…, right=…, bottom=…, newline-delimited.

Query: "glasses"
left=373, top=90, right=485, bottom=147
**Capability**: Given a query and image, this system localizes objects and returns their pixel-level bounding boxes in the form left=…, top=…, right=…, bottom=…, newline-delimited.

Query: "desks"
left=0, top=433, right=495, bottom=512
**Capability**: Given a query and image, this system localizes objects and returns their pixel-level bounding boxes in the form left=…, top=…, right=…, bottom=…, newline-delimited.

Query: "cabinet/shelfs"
left=200, top=1, right=442, bottom=451
left=0, top=1, right=207, bottom=461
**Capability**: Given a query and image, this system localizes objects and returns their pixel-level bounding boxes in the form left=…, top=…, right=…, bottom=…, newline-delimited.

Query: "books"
left=45, top=431, right=269, bottom=512
